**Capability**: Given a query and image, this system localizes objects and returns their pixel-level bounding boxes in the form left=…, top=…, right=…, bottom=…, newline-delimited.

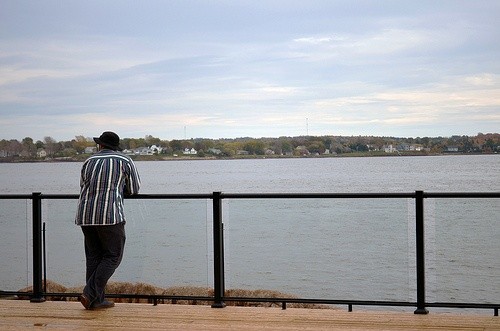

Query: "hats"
left=93, top=132, right=123, bottom=150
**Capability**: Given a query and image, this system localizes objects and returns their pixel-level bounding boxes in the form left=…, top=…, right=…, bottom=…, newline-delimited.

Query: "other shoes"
left=81, top=294, right=92, bottom=310
left=93, top=301, right=115, bottom=308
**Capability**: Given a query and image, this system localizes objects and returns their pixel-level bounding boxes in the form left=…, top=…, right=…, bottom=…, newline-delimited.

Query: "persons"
left=75, top=131, right=141, bottom=307
